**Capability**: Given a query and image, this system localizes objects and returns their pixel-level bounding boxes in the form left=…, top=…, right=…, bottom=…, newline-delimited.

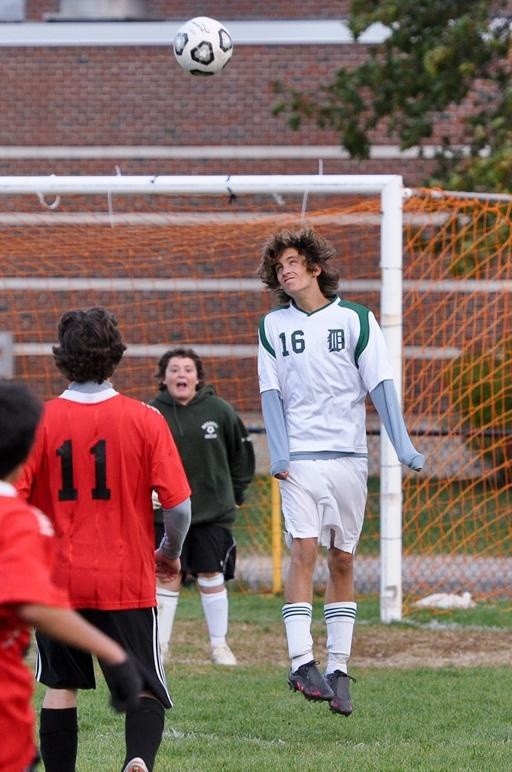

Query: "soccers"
left=174, top=17, right=234, bottom=75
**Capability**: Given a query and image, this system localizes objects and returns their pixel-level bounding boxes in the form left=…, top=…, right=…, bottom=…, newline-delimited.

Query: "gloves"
left=108, top=658, right=139, bottom=713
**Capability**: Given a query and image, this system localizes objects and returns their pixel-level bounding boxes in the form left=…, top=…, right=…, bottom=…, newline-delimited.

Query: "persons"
left=19, top=306, right=193, bottom=772
left=0, top=379, right=142, bottom=771
left=150, top=347, right=256, bottom=666
left=258, top=226, right=420, bottom=715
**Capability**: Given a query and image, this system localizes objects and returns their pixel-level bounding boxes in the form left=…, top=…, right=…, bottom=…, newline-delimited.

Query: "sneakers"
left=212, top=645, right=236, bottom=666
left=288, top=660, right=335, bottom=703
left=324, top=670, right=356, bottom=718
left=120, top=754, right=152, bottom=772
